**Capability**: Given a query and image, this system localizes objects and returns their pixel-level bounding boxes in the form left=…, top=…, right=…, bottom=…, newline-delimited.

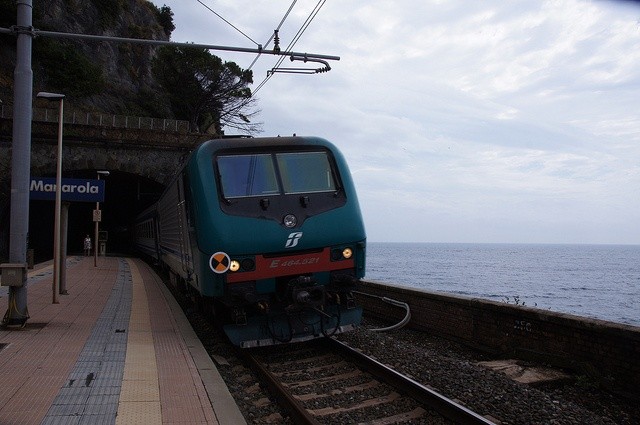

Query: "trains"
left=131, top=137, right=367, bottom=349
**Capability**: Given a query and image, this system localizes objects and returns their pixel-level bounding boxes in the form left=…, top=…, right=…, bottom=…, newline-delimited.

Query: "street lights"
left=95, top=171, right=109, bottom=266
left=37, top=92, right=65, bottom=303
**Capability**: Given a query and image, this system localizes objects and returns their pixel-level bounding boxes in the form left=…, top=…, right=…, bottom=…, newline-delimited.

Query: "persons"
left=84, top=233, right=91, bottom=257
